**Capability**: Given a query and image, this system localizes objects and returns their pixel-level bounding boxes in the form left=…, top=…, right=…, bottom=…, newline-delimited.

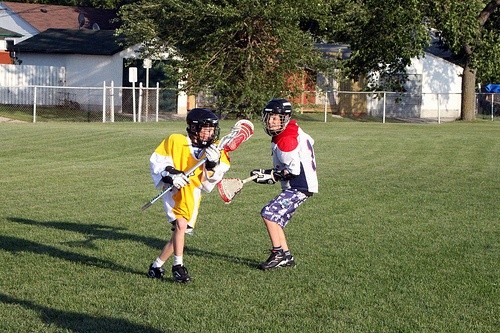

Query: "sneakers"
left=274, top=254, right=296, bottom=268
left=172, top=264, right=192, bottom=284
left=147, top=263, right=166, bottom=281
left=257, top=247, right=288, bottom=269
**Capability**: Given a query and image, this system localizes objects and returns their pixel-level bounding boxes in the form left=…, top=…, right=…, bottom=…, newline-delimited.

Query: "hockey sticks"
left=217, top=173, right=259, bottom=203
left=141, top=118, right=255, bottom=212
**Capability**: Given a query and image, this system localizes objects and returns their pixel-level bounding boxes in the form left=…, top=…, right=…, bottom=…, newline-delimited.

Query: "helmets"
left=186, top=107, right=220, bottom=148
left=262, top=99, right=292, bottom=137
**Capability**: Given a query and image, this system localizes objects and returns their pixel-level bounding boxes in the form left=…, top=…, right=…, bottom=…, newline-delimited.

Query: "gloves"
left=204, top=145, right=221, bottom=171
left=250, top=168, right=278, bottom=185
left=162, top=166, right=191, bottom=189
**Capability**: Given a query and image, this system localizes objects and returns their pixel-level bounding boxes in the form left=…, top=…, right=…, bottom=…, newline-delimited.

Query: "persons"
left=251, top=98, right=318, bottom=270
left=147, top=108, right=231, bottom=284
